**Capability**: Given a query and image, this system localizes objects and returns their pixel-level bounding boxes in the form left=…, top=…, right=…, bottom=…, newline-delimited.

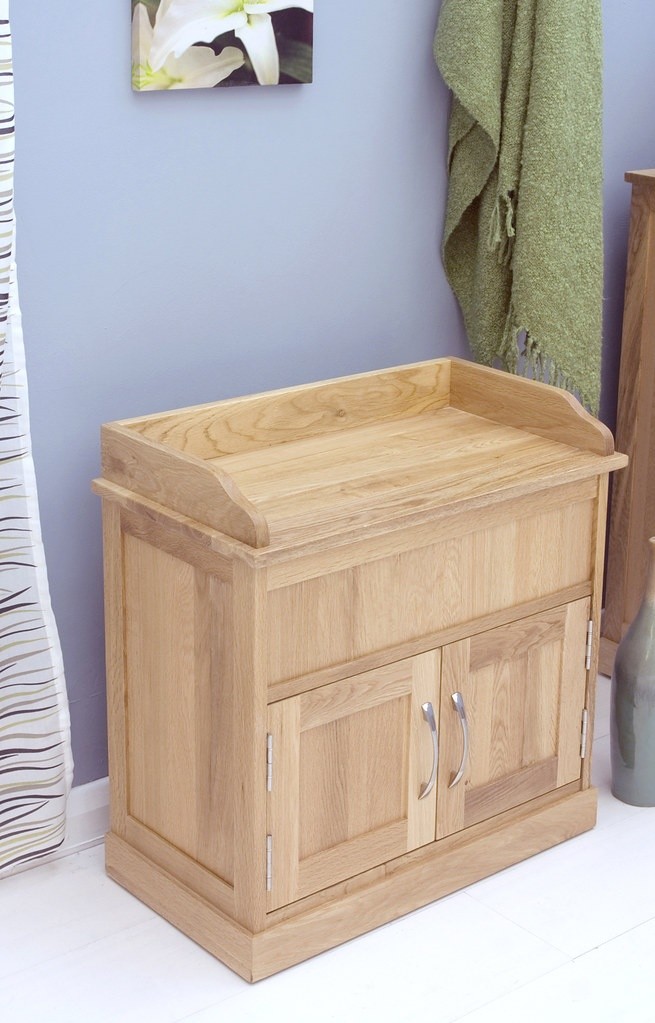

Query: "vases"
left=609, top=535, right=655, bottom=807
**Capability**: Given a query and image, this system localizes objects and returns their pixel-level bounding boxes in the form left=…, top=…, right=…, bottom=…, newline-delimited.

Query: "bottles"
left=604, top=535, right=655, bottom=808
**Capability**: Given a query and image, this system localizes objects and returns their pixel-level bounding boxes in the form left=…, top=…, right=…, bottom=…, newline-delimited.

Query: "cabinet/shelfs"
left=91, top=356, right=629, bottom=984
